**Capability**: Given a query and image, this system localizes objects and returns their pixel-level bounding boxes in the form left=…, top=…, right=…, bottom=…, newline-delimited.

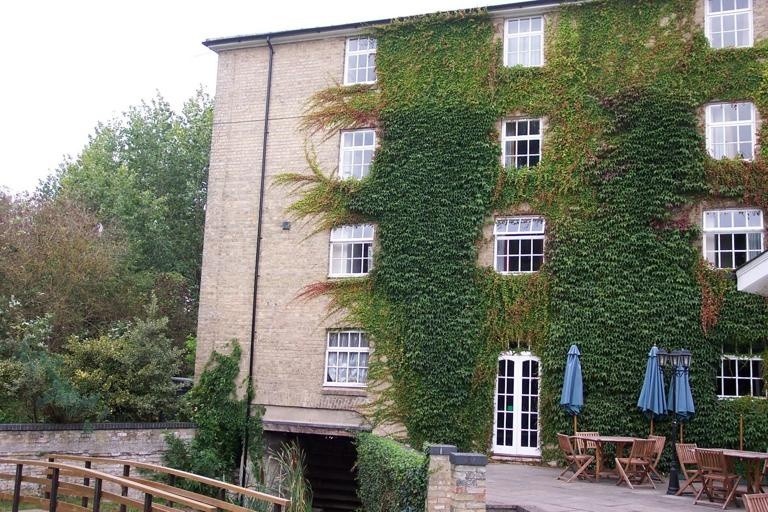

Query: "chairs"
left=555, top=432, right=768, bottom=512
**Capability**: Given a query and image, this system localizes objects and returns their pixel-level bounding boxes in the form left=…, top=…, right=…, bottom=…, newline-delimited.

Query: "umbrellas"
left=558, top=345, right=584, bottom=449
left=636, top=342, right=666, bottom=435
left=666, top=348, right=695, bottom=444
